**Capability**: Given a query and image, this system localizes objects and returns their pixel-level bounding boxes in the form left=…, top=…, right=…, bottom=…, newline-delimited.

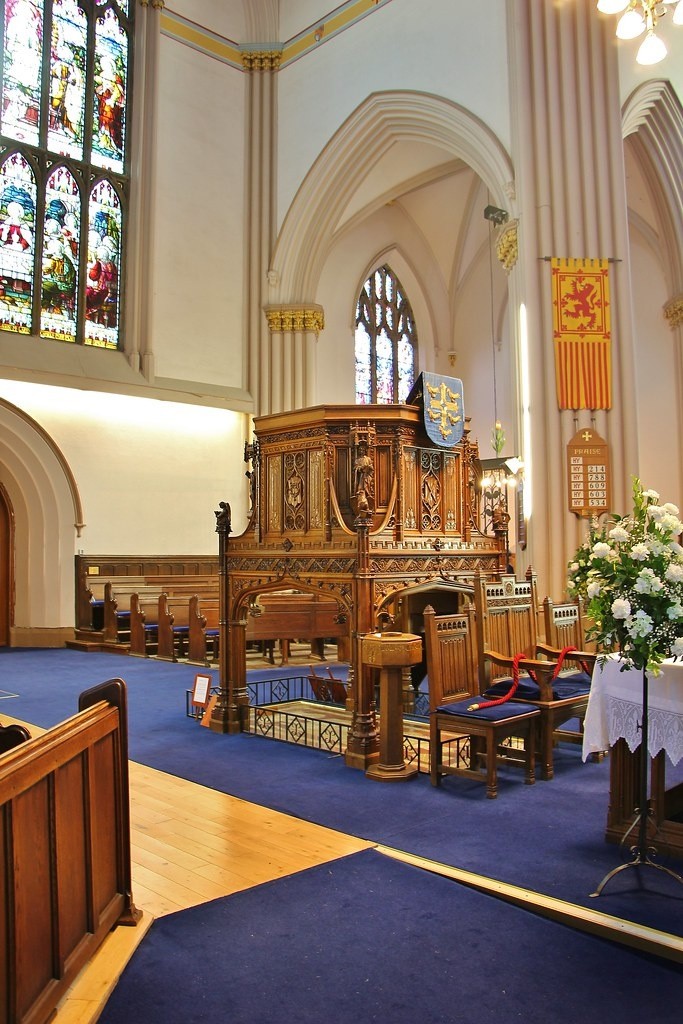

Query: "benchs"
left=91, top=583, right=346, bottom=665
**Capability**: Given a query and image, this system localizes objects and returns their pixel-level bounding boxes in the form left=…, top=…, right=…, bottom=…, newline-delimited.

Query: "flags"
left=550, top=258, right=612, bottom=412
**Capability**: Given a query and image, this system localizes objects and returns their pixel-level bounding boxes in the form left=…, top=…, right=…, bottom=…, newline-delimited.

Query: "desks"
left=582, top=648, right=683, bottom=867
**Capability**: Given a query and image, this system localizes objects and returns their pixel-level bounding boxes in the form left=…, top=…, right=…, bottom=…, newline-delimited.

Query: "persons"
left=353, top=446, right=375, bottom=511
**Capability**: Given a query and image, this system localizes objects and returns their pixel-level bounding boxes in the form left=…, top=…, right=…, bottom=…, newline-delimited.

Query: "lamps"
left=481, top=457, right=527, bottom=534
left=597, top=0, right=683, bottom=65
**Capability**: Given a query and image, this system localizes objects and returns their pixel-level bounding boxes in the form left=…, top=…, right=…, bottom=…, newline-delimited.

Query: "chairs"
left=423, top=564, right=603, bottom=798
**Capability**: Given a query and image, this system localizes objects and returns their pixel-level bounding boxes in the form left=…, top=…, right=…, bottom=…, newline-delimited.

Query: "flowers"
left=566, top=475, right=683, bottom=679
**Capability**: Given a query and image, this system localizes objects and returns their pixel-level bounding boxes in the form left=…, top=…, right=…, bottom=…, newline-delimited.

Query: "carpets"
left=0, top=647, right=682, bottom=935
left=91, top=844, right=683, bottom=1024
